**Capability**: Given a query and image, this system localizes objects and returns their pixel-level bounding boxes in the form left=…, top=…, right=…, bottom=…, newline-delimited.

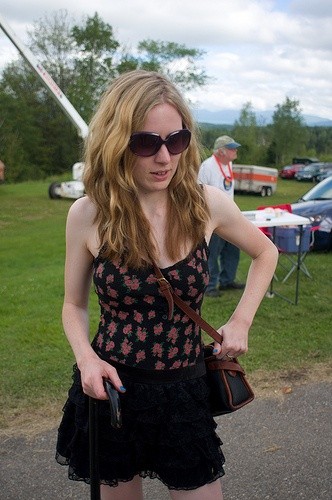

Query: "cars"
left=280, top=164, right=306, bottom=179
left=295, top=163, right=328, bottom=182
left=316, top=161, right=332, bottom=181
left=261, top=175, right=332, bottom=250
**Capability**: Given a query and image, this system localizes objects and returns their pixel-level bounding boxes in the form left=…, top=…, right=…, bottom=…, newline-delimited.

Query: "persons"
left=196, top=135, right=245, bottom=297
left=62, top=69, right=280, bottom=500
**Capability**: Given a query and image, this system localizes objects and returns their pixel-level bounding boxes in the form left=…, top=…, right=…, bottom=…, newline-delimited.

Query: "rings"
left=226, top=353, right=233, bottom=359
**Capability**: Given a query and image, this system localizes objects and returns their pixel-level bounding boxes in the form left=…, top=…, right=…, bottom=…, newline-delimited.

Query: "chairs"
left=257, top=204, right=320, bottom=285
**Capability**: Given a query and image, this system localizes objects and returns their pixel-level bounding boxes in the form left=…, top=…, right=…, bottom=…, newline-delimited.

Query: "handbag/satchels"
left=203, top=342, right=255, bottom=417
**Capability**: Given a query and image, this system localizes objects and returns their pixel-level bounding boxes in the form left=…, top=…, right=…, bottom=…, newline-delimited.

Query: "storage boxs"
left=269, top=225, right=312, bottom=253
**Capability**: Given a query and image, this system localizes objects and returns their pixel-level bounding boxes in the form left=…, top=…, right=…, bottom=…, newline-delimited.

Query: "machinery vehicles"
left=0, top=18, right=95, bottom=199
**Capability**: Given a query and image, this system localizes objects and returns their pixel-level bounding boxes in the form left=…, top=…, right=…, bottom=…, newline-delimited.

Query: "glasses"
left=128, top=120, right=191, bottom=157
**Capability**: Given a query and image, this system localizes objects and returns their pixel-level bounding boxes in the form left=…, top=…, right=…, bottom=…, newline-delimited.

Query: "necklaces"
left=214, top=153, right=235, bottom=182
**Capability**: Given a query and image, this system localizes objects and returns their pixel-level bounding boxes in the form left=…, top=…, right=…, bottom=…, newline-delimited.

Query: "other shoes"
left=219, top=281, right=244, bottom=290
left=205, top=289, right=221, bottom=297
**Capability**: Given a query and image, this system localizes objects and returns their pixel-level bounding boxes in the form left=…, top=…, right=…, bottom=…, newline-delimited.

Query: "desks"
left=240, top=211, right=310, bottom=306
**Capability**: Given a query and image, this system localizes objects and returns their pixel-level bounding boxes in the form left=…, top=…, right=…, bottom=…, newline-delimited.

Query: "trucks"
left=293, top=159, right=313, bottom=166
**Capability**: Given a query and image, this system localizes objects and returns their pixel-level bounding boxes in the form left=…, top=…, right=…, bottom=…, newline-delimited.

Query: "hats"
left=214, top=135, right=242, bottom=150
